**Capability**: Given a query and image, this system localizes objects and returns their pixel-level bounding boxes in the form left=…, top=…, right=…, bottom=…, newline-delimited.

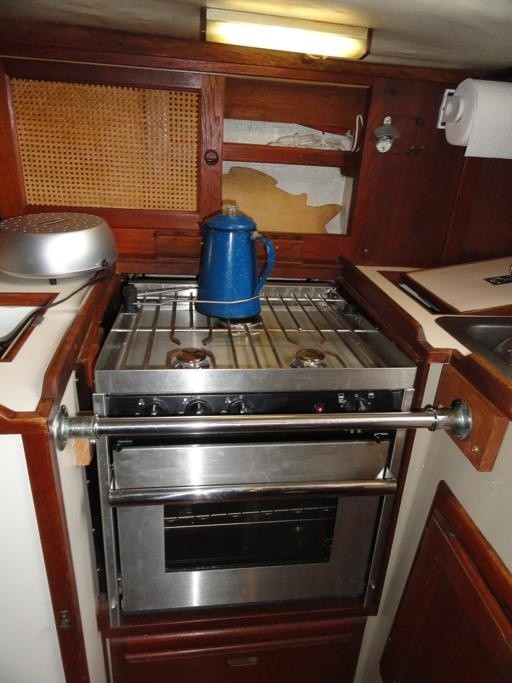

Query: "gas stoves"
left=94, top=273, right=412, bottom=442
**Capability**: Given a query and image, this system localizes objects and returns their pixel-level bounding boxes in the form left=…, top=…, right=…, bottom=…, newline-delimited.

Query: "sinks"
left=435, top=317, right=512, bottom=380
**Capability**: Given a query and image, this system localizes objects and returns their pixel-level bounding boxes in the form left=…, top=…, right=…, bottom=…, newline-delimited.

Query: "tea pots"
left=195, top=201, right=274, bottom=318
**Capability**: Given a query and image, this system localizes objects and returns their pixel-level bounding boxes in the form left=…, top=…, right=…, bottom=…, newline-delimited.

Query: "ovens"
left=96, top=440, right=401, bottom=628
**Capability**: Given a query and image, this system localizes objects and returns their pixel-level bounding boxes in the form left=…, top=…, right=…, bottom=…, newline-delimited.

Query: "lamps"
left=196, top=6, right=373, bottom=61
